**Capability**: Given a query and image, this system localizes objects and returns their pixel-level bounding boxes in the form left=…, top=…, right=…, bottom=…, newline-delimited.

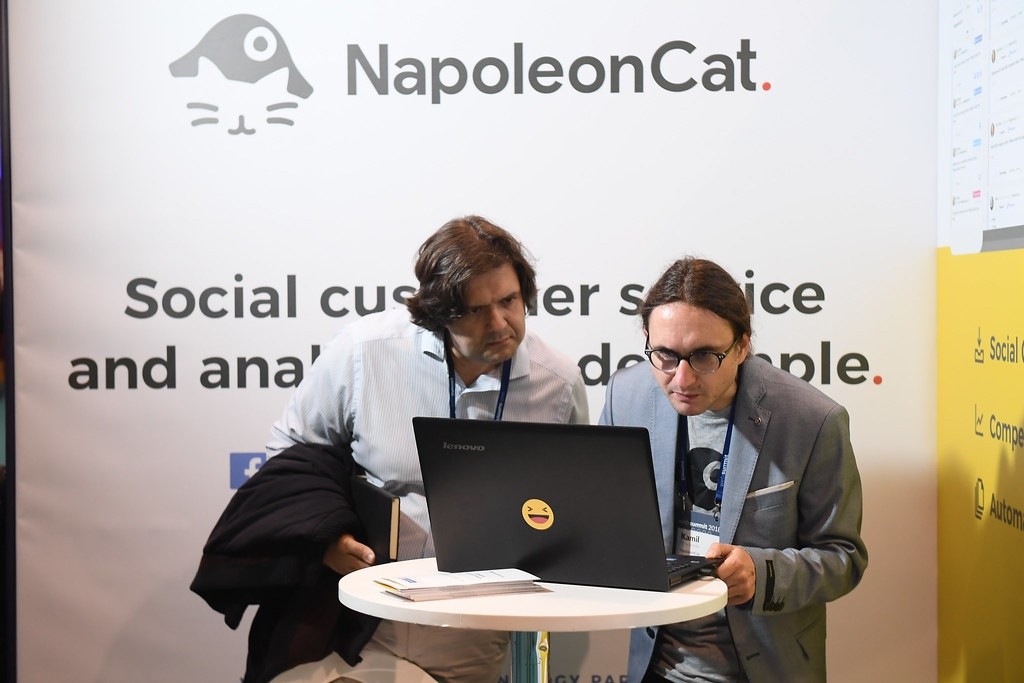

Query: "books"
left=351, top=476, right=401, bottom=561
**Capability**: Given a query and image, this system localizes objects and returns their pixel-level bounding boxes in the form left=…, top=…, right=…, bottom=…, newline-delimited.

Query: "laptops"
left=411, top=416, right=723, bottom=592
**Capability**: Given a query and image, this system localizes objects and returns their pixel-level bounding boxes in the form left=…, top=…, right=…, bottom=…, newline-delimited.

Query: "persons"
left=265, top=215, right=590, bottom=683
left=599, top=259, right=870, bottom=683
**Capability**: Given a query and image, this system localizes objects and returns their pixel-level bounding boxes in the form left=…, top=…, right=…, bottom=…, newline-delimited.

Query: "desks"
left=339, top=558, right=728, bottom=683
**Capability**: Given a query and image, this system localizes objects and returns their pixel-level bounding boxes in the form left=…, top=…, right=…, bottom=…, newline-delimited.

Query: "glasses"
left=645, top=332, right=739, bottom=374
